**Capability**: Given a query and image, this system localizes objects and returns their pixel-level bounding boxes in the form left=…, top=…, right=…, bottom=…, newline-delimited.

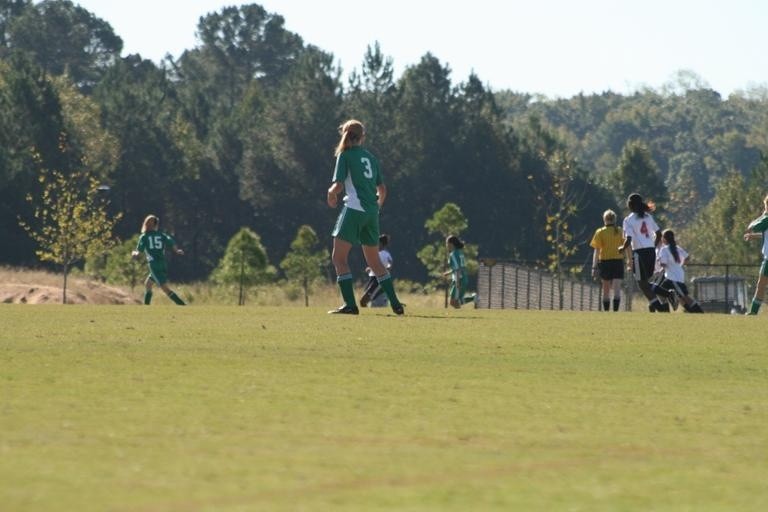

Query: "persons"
left=743, top=195, right=768, bottom=315
left=442, top=235, right=481, bottom=310
left=327, top=120, right=407, bottom=314
left=589, top=208, right=632, bottom=312
left=653, top=232, right=692, bottom=313
left=617, top=193, right=679, bottom=313
left=360, top=234, right=392, bottom=307
left=132, top=215, right=186, bottom=305
left=660, top=229, right=704, bottom=312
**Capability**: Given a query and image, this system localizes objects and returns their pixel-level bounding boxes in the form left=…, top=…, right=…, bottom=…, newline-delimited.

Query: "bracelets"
left=591, top=267, right=598, bottom=270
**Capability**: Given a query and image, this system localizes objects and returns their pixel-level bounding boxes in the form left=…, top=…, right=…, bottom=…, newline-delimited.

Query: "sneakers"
left=472, top=292, right=481, bottom=309
left=393, top=303, right=406, bottom=316
left=666, top=287, right=680, bottom=312
left=326, top=303, right=360, bottom=315
